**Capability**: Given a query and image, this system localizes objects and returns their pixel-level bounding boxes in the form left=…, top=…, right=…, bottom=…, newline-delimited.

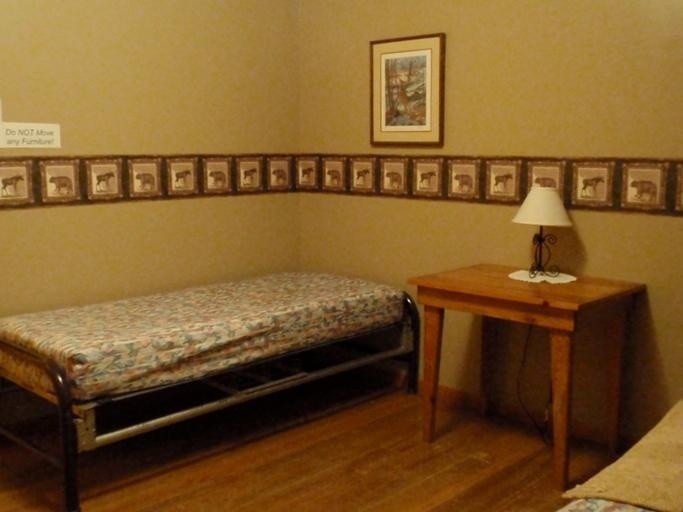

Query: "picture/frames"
left=294, top=153, right=321, bottom=194
left=36, top=155, right=83, bottom=205
left=201, top=154, right=233, bottom=196
left=669, top=159, right=681, bottom=214
left=321, top=154, right=347, bottom=194
left=265, top=154, right=294, bottom=193
left=369, top=33, right=446, bottom=147
left=378, top=153, right=411, bottom=198
left=567, top=156, right=616, bottom=210
left=165, top=153, right=201, bottom=199
left=411, top=155, right=445, bottom=200
left=83, top=154, right=125, bottom=203
left=525, top=158, right=568, bottom=207
left=485, top=156, right=524, bottom=205
left=125, top=154, right=165, bottom=201
left=445, top=155, right=482, bottom=203
left=349, top=154, right=378, bottom=195
left=617, top=155, right=666, bottom=214
left=234, top=154, right=264, bottom=194
left=0, top=155, right=37, bottom=209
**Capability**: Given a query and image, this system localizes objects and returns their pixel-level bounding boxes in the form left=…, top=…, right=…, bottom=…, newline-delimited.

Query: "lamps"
left=510, top=186, right=573, bottom=278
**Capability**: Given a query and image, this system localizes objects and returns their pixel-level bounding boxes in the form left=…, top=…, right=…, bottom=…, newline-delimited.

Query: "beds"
left=1, top=272, right=419, bottom=511
left=553, top=398, right=682, bottom=511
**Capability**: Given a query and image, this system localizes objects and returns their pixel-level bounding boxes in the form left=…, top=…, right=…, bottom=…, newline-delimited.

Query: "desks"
left=406, top=262, right=646, bottom=493
